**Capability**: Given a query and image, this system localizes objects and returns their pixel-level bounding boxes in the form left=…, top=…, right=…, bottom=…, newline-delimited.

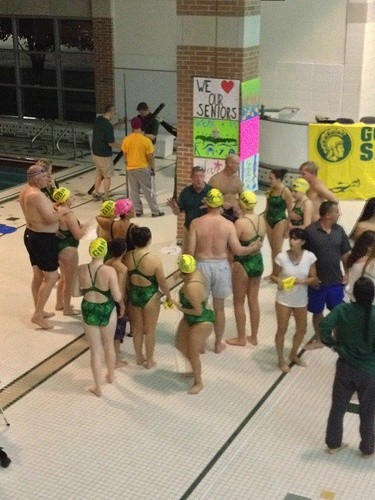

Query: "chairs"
left=276, top=108, right=299, bottom=121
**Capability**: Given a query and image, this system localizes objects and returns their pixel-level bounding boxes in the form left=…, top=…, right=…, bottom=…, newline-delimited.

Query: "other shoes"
left=151, top=211, right=165, bottom=217
left=136, top=213, right=142, bottom=217
left=90, top=191, right=110, bottom=202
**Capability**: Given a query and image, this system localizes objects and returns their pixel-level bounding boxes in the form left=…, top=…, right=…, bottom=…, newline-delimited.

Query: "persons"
left=121, top=118, right=166, bottom=217
left=260, top=159, right=338, bottom=282
left=167, top=165, right=217, bottom=231
left=135, top=101, right=159, bottom=145
left=91, top=104, right=122, bottom=202
left=318, top=276, right=375, bottom=456
left=227, top=190, right=266, bottom=347
left=99, top=239, right=130, bottom=367
left=207, top=153, right=245, bottom=224
left=182, top=189, right=263, bottom=354
left=166, top=253, right=216, bottom=395
left=36, top=158, right=59, bottom=201
left=19, top=165, right=75, bottom=332
left=301, top=199, right=351, bottom=340
left=121, top=225, right=172, bottom=369
left=72, top=236, right=121, bottom=396
left=345, top=195, right=375, bottom=308
left=52, top=186, right=91, bottom=316
left=94, top=197, right=137, bottom=243
left=270, top=227, right=318, bottom=372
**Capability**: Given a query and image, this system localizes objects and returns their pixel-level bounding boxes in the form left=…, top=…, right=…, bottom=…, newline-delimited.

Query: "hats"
left=205, top=187, right=224, bottom=208
left=115, top=198, right=133, bottom=214
left=281, top=277, right=295, bottom=291
left=238, top=191, right=257, bottom=210
left=131, top=117, right=142, bottom=130
left=290, top=178, right=311, bottom=193
left=52, top=186, right=71, bottom=203
left=178, top=253, right=196, bottom=274
left=100, top=200, right=116, bottom=217
left=88, top=238, right=108, bottom=260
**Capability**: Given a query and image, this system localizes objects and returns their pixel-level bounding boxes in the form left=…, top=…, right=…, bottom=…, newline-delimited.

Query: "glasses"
left=290, top=183, right=300, bottom=188
left=28, top=166, right=47, bottom=177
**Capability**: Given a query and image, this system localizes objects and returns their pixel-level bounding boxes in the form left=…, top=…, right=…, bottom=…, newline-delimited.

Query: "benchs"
left=84, top=128, right=176, bottom=158
